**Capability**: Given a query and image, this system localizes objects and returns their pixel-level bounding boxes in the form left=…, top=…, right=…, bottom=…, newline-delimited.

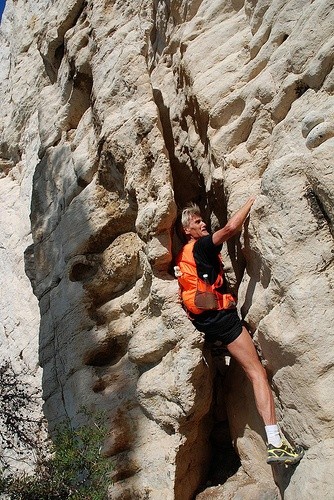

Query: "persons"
left=168, top=192, right=304, bottom=466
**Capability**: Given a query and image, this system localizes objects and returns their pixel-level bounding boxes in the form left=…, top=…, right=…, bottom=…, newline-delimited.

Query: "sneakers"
left=265, top=433, right=304, bottom=465
